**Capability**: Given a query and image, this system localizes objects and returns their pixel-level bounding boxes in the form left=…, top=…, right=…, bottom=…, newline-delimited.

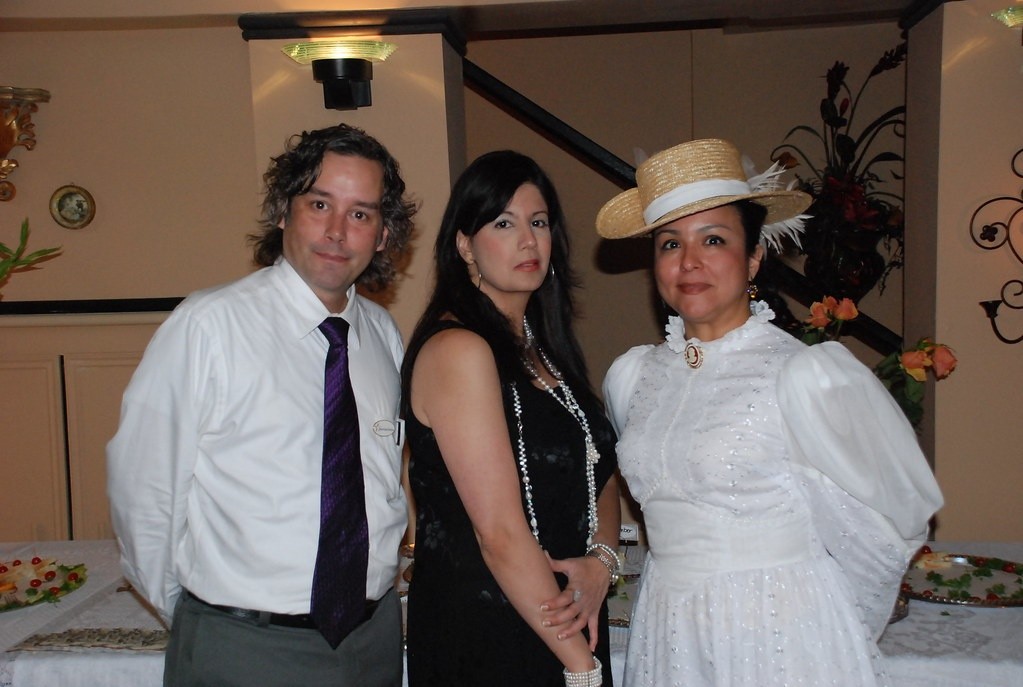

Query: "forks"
left=4, top=594, right=22, bottom=606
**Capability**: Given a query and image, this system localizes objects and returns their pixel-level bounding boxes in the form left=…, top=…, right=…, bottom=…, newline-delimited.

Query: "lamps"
left=281, top=39, right=399, bottom=111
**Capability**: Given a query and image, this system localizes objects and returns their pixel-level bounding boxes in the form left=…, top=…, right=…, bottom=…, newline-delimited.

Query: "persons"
left=596, top=138, right=945, bottom=687
left=108, top=122, right=416, bottom=687
left=402, top=150, right=623, bottom=687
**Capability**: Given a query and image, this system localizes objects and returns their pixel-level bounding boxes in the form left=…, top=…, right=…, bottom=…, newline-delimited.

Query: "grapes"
left=0, top=557, right=78, bottom=594
left=900, top=546, right=1015, bottom=601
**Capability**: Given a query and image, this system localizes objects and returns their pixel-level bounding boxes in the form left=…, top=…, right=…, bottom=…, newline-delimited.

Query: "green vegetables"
left=0, top=562, right=87, bottom=614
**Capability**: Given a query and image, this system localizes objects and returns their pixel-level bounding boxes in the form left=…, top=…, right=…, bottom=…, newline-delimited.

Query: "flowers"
left=769, top=42, right=908, bottom=308
left=800, top=295, right=957, bottom=436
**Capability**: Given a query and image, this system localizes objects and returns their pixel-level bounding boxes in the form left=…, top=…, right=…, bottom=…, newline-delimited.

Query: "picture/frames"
left=49, top=184, right=101, bottom=229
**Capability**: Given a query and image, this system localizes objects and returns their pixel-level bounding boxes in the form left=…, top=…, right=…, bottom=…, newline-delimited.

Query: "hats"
left=596, top=138, right=815, bottom=261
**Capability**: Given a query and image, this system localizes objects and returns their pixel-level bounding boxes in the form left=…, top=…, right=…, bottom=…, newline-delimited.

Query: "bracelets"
left=563, top=655, right=603, bottom=687
left=586, top=543, right=620, bottom=571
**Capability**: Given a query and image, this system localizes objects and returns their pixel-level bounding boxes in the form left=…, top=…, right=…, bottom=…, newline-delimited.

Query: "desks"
left=0, top=540, right=1023, bottom=687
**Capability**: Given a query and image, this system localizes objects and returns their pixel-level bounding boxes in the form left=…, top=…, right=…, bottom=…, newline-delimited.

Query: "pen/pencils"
left=395, top=418, right=405, bottom=448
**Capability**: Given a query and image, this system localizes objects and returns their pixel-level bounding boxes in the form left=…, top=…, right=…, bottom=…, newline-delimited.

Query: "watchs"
left=585, top=550, right=620, bottom=585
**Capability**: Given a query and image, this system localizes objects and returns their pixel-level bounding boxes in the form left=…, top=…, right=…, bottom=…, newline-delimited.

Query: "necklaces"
left=509, top=312, right=600, bottom=556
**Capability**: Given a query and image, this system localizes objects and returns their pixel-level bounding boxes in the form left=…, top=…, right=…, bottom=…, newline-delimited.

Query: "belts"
left=182, top=586, right=395, bottom=631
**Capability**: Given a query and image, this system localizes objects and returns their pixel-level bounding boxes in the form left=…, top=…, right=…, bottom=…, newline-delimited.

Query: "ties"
left=309, top=316, right=369, bottom=651
